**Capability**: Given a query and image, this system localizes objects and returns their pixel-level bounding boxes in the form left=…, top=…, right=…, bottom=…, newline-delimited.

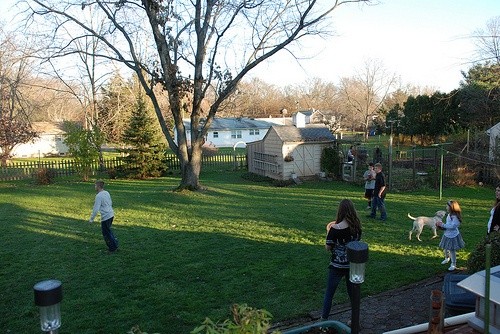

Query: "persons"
left=89, top=180, right=119, bottom=253
left=347, top=143, right=388, bottom=220
left=435, top=200, right=466, bottom=271
left=319, top=199, right=362, bottom=332
left=486, top=185, right=500, bottom=235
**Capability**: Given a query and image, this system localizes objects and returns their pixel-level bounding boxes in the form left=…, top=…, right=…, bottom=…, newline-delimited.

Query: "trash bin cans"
left=369, top=130, right=376, bottom=136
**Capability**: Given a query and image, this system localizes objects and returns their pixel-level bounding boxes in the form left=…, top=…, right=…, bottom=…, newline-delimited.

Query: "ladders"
left=252, top=151, right=279, bottom=174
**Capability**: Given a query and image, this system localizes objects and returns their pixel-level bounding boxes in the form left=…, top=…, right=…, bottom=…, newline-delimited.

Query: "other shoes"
left=441, top=259, right=450, bottom=264
left=366, top=214, right=375, bottom=218
left=347, top=320, right=352, bottom=327
left=448, top=265, right=457, bottom=271
left=102, top=249, right=118, bottom=254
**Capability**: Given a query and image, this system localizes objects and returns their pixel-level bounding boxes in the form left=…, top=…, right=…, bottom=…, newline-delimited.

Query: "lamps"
left=33, top=279, right=65, bottom=332
left=345, top=238, right=369, bottom=291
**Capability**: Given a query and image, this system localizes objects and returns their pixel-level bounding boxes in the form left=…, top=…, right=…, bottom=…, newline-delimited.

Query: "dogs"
left=408, top=210, right=446, bottom=242
